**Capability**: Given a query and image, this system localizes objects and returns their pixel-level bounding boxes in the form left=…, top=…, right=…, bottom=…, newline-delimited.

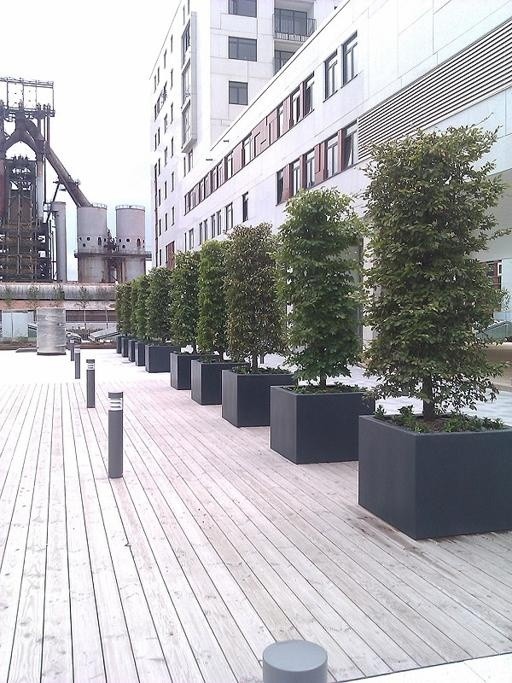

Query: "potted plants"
left=115, top=265, right=181, bottom=375
left=265, top=183, right=376, bottom=464
left=221, top=210, right=296, bottom=430
left=168, top=248, right=221, bottom=392
left=190, top=235, right=251, bottom=406
left=355, top=133, right=512, bottom=537
